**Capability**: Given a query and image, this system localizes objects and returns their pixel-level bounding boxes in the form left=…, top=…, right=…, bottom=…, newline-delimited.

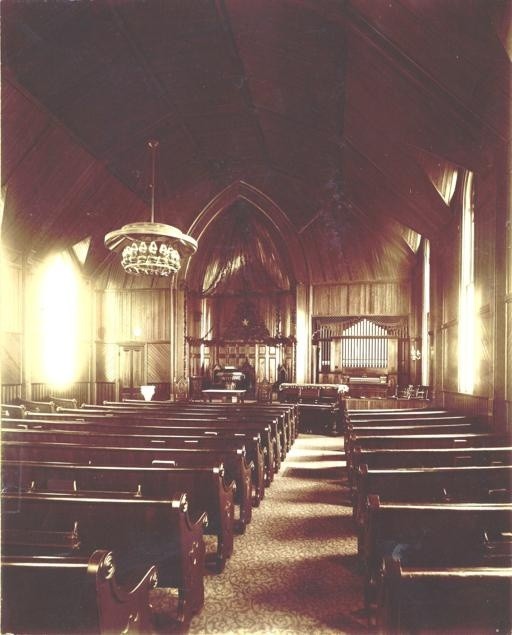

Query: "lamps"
left=103, top=137, right=199, bottom=278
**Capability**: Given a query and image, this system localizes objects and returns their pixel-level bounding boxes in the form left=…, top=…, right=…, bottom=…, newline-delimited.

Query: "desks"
left=201, top=388, right=246, bottom=404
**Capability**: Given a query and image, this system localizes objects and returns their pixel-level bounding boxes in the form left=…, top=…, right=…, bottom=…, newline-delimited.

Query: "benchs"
left=342, top=406, right=512, bottom=635
